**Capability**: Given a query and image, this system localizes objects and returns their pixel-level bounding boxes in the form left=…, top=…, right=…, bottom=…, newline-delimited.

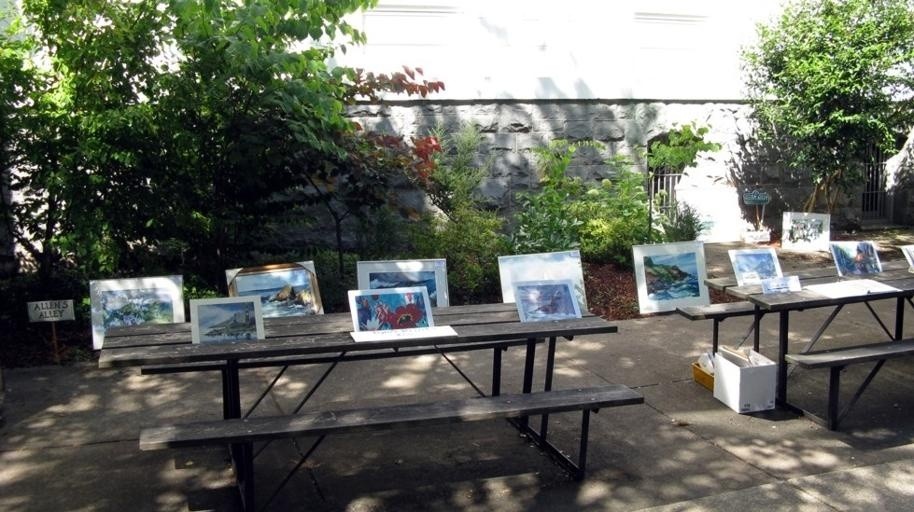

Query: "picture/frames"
left=512, top=280, right=583, bottom=323
left=729, top=247, right=783, bottom=288
left=90, top=275, right=186, bottom=351
left=189, top=295, right=265, bottom=345
left=899, top=245, right=914, bottom=274
left=632, top=241, right=710, bottom=315
left=828, top=239, right=884, bottom=277
left=782, top=212, right=831, bottom=257
left=346, top=285, right=435, bottom=333
left=356, top=258, right=450, bottom=309
left=224, top=260, right=324, bottom=319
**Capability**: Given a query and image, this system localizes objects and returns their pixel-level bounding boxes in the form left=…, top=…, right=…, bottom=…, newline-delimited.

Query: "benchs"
left=140, top=384, right=644, bottom=512
left=141, top=337, right=544, bottom=413
left=784, top=338, right=914, bottom=431
left=676, top=297, right=769, bottom=356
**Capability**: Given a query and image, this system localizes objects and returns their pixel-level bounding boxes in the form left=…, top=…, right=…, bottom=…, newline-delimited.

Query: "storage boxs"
left=712, top=347, right=776, bottom=415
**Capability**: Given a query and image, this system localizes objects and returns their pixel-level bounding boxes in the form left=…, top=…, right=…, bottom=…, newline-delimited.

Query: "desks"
left=98, top=303, right=617, bottom=511
left=704, top=258, right=914, bottom=431
